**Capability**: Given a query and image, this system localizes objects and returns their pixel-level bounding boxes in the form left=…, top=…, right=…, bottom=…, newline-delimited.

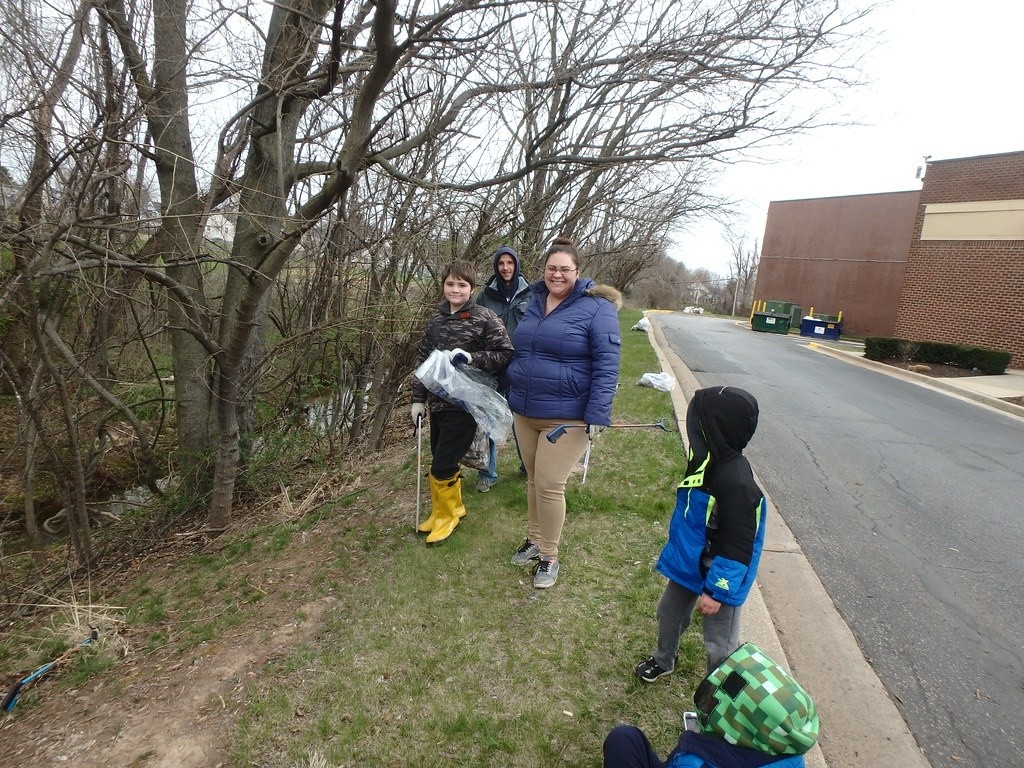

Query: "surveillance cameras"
left=923, top=155, right=931, bottom=158
left=916, top=166, right=922, bottom=178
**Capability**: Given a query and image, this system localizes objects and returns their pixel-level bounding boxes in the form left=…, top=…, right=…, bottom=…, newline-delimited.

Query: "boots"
left=426, top=474, right=461, bottom=544
left=417, top=468, right=466, bottom=533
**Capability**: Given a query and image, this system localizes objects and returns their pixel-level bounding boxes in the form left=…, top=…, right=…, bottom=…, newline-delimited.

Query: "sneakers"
left=636, top=656, right=675, bottom=682
left=475, top=477, right=496, bottom=492
left=510, top=538, right=539, bottom=566
left=532, top=555, right=559, bottom=589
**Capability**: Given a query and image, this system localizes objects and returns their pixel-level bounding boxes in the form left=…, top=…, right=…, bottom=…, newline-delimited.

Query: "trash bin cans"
left=800, top=316, right=842, bottom=340
left=765, top=300, right=843, bottom=328
left=752, top=312, right=791, bottom=335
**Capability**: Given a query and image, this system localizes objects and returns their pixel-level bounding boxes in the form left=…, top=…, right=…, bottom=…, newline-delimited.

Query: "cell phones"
left=683, top=711, right=703, bottom=735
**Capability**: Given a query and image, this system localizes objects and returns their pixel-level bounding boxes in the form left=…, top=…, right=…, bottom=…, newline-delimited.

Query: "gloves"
left=412, top=402, right=425, bottom=426
left=449, top=348, right=473, bottom=368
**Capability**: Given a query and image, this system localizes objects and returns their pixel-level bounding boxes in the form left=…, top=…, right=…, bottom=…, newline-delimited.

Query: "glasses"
left=544, top=264, right=577, bottom=274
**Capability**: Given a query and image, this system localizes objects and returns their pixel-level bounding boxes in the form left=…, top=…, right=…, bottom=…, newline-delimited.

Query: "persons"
left=603, top=644, right=819, bottom=768
left=475, top=247, right=531, bottom=491
left=636, top=387, right=766, bottom=683
left=409, top=258, right=514, bottom=552
left=501, top=238, right=621, bottom=589
